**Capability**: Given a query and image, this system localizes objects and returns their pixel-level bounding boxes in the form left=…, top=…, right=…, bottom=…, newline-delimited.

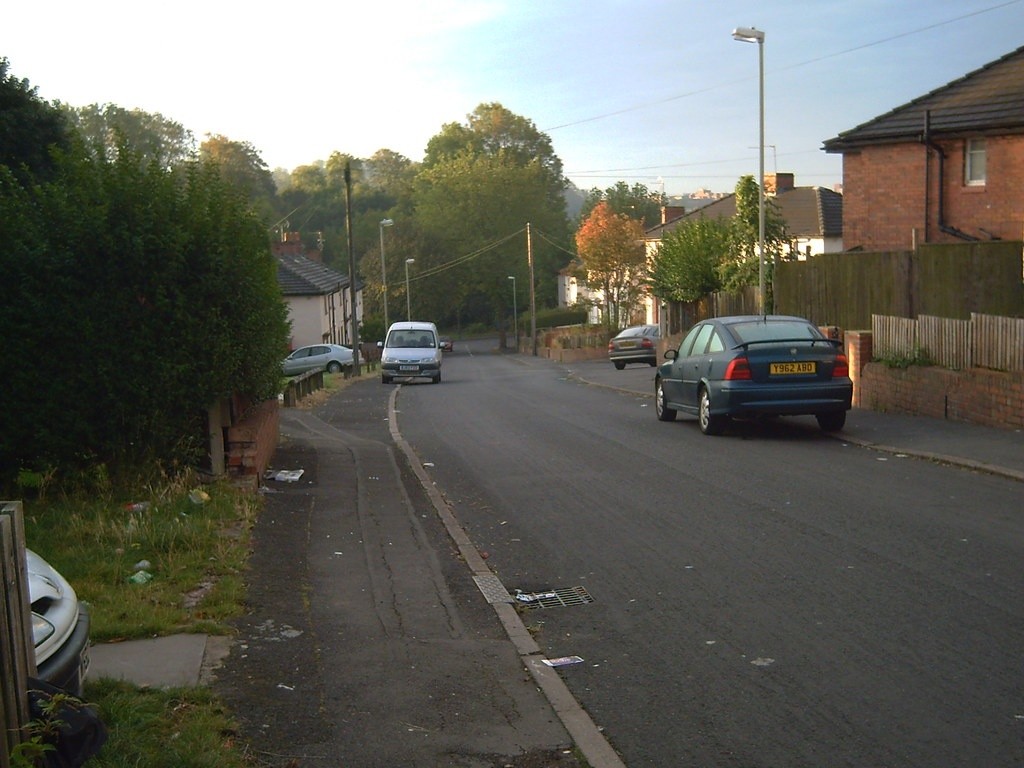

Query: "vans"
left=377, top=321, right=447, bottom=384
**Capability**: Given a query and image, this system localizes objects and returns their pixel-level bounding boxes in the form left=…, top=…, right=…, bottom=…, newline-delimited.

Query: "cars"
left=25, top=548, right=92, bottom=697
left=439, top=338, right=454, bottom=352
left=274, top=344, right=364, bottom=378
left=608, top=324, right=656, bottom=370
left=654, top=315, right=854, bottom=437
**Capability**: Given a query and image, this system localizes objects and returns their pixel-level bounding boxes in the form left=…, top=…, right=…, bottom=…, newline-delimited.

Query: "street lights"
left=404, top=254, right=416, bottom=322
left=380, top=219, right=395, bottom=336
left=733, top=25, right=767, bottom=314
left=508, top=276, right=516, bottom=354
